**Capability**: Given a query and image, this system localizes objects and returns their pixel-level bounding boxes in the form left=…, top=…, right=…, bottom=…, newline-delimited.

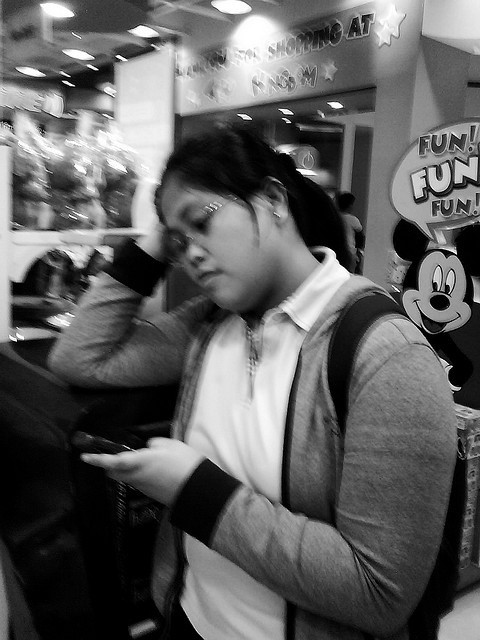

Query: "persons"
left=336, top=193, right=363, bottom=274
left=45, top=124, right=458, bottom=640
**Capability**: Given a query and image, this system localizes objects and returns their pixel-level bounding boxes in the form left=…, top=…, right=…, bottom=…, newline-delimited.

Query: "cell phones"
left=71, top=428, right=143, bottom=458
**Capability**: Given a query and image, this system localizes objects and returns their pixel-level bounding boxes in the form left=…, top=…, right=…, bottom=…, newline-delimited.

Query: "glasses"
left=169, top=196, right=236, bottom=264
